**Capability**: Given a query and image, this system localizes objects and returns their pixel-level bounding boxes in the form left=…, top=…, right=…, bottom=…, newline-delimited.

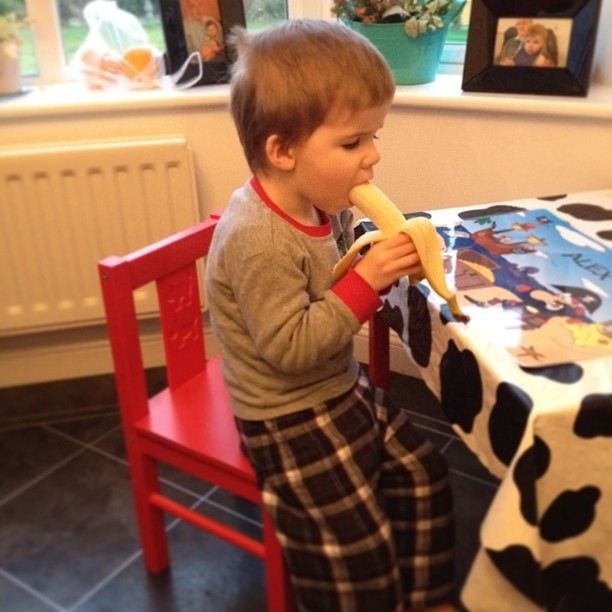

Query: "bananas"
left=331, top=181, right=462, bottom=318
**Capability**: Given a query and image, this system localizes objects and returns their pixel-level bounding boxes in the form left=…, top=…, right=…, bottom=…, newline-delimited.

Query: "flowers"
left=330, top=0, right=451, bottom=38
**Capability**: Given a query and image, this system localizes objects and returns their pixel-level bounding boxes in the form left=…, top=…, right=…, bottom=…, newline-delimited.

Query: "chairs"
left=96, top=211, right=295, bottom=612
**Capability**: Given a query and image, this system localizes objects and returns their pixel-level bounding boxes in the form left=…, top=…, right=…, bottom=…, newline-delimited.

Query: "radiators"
left=0, top=137, right=209, bottom=338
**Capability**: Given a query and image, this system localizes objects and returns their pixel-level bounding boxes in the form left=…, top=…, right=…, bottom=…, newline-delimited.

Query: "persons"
left=500, top=18, right=534, bottom=60
left=181, top=1, right=223, bottom=62
left=204, top=18, right=461, bottom=612
left=201, top=20, right=226, bottom=63
left=500, top=24, right=554, bottom=66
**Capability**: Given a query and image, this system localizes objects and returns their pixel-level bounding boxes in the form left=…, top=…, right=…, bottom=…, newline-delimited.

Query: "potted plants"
left=0, top=10, right=28, bottom=97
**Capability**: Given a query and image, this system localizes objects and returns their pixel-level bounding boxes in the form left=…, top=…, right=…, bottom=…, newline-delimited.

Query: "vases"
left=338, top=0, right=466, bottom=85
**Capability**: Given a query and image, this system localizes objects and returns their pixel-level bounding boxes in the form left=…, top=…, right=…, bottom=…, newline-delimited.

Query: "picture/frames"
left=461, top=0, right=603, bottom=100
left=159, top=0, right=248, bottom=90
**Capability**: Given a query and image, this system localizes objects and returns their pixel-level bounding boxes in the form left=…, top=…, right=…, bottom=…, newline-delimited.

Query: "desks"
left=355, top=189, right=610, bottom=611
